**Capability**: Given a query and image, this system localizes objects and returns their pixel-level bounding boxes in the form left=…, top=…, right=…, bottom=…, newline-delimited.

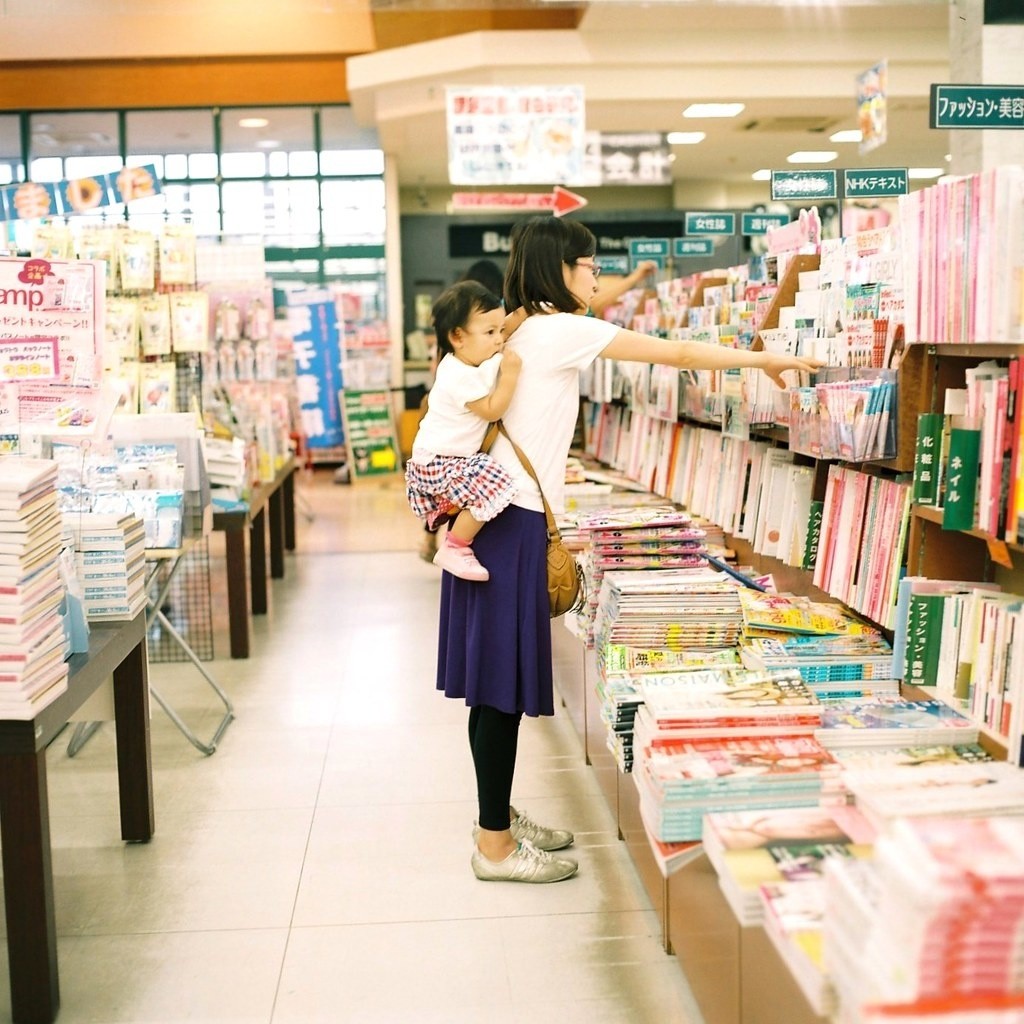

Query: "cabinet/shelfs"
left=552, top=256, right=1023, bottom=1022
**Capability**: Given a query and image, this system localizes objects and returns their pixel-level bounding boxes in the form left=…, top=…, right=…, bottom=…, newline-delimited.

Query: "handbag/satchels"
left=547, top=536, right=587, bottom=617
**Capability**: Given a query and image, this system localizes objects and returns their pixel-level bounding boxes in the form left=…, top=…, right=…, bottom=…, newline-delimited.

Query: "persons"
left=405, top=216, right=826, bottom=883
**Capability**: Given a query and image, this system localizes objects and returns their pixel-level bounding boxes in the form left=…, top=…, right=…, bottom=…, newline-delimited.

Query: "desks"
left=1, top=613, right=152, bottom=1023
left=210, top=453, right=297, bottom=659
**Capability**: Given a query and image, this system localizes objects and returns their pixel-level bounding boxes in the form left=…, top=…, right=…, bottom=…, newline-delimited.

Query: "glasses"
left=575, top=263, right=603, bottom=278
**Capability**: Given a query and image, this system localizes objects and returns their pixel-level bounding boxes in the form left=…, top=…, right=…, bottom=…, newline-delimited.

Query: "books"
left=0, top=454, right=150, bottom=720
left=558, top=166, right=1024, bottom=1024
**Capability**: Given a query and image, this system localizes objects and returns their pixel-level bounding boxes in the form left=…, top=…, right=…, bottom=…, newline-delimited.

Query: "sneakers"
left=432, top=540, right=490, bottom=582
left=420, top=539, right=436, bottom=561
left=471, top=805, right=580, bottom=884
